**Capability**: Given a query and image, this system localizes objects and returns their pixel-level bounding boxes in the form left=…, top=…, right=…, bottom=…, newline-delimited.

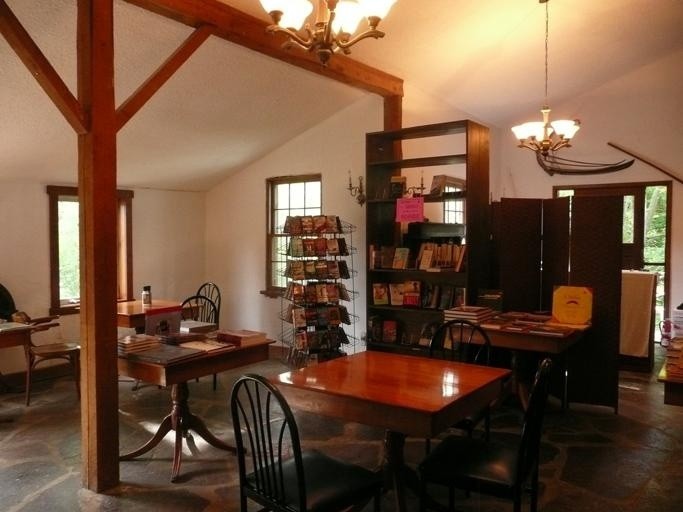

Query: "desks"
left=268, top=350, right=513, bottom=510
left=657, top=337, right=683, bottom=407
left=0, top=318, right=38, bottom=348
left=117, top=328, right=276, bottom=483
left=74, top=299, right=203, bottom=333
left=444, top=313, right=578, bottom=411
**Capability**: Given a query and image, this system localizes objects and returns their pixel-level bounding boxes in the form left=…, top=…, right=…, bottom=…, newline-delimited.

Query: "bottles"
left=140, top=285, right=152, bottom=308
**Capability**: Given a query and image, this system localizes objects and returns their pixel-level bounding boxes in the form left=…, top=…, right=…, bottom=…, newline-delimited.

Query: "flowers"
left=260, top=0, right=398, bottom=69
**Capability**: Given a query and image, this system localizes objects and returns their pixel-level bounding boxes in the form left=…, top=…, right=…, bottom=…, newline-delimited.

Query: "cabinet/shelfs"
left=275, top=221, right=361, bottom=370
left=366, top=120, right=490, bottom=430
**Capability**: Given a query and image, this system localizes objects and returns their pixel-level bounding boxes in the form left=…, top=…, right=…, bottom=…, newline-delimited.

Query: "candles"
left=348, top=169, right=351, bottom=184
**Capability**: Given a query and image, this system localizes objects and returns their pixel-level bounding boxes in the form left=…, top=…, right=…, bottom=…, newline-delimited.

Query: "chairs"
left=13, top=310, right=82, bottom=407
left=418, top=358, right=552, bottom=511
left=425, top=317, right=495, bottom=510
left=130, top=282, right=221, bottom=390
left=230, top=374, right=382, bottom=511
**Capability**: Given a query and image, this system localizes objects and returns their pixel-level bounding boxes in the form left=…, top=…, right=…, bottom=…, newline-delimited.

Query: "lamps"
left=511, top=1, right=581, bottom=162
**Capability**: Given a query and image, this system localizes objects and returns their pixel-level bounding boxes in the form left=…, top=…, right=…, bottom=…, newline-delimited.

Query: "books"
left=663, top=336, right=683, bottom=381
left=466, top=301, right=577, bottom=340
left=368, top=238, right=466, bottom=350
left=389, top=174, right=406, bottom=201
left=280, top=214, right=351, bottom=370
left=117, top=317, right=267, bottom=364
